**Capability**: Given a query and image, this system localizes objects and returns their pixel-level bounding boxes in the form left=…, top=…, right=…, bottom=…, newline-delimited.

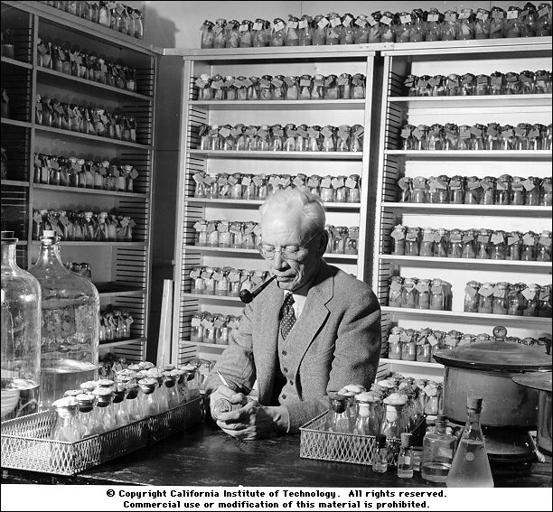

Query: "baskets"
left=1, top=395, right=205, bottom=475
left=299, top=408, right=425, bottom=466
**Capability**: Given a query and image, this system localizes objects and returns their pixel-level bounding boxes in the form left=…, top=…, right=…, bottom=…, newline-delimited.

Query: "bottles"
left=186, top=309, right=552, bottom=371
left=49, top=366, right=203, bottom=467
left=211, top=397, right=244, bottom=418
left=198, top=122, right=553, bottom=156
left=318, top=372, right=496, bottom=486
left=194, top=172, right=552, bottom=206
left=197, top=1, right=552, bottom=47
left=193, top=219, right=553, bottom=263
left=194, top=68, right=553, bottom=101
left=32, top=0, right=149, bottom=347
left=188, top=266, right=552, bottom=319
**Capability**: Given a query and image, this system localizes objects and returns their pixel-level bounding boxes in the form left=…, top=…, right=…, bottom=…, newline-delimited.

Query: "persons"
left=203, top=189, right=383, bottom=442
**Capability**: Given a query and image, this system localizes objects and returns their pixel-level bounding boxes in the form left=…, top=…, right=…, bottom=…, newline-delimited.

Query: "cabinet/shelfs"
left=30, top=7, right=160, bottom=403
left=163, top=42, right=373, bottom=393
left=370, top=36, right=551, bottom=398
left=1, top=1, right=32, bottom=274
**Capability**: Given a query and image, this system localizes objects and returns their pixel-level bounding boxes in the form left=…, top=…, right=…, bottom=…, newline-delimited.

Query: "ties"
left=279, top=296, right=296, bottom=339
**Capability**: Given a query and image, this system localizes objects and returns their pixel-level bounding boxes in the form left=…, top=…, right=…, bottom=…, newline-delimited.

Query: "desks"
left=0, top=394, right=553, bottom=486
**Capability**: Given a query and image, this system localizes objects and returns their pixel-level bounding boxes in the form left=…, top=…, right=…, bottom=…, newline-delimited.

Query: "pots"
left=429, top=323, right=553, bottom=458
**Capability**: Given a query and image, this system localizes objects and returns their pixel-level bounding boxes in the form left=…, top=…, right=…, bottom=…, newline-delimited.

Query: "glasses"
left=257, top=243, right=302, bottom=261
